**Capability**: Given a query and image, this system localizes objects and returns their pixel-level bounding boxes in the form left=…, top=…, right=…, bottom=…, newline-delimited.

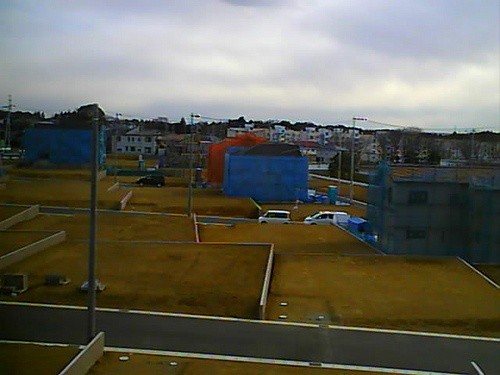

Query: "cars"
left=138, top=173, right=165, bottom=188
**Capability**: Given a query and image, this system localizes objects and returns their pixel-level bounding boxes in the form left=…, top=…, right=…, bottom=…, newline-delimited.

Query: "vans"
left=258, top=209, right=291, bottom=223
left=303, top=211, right=350, bottom=225
